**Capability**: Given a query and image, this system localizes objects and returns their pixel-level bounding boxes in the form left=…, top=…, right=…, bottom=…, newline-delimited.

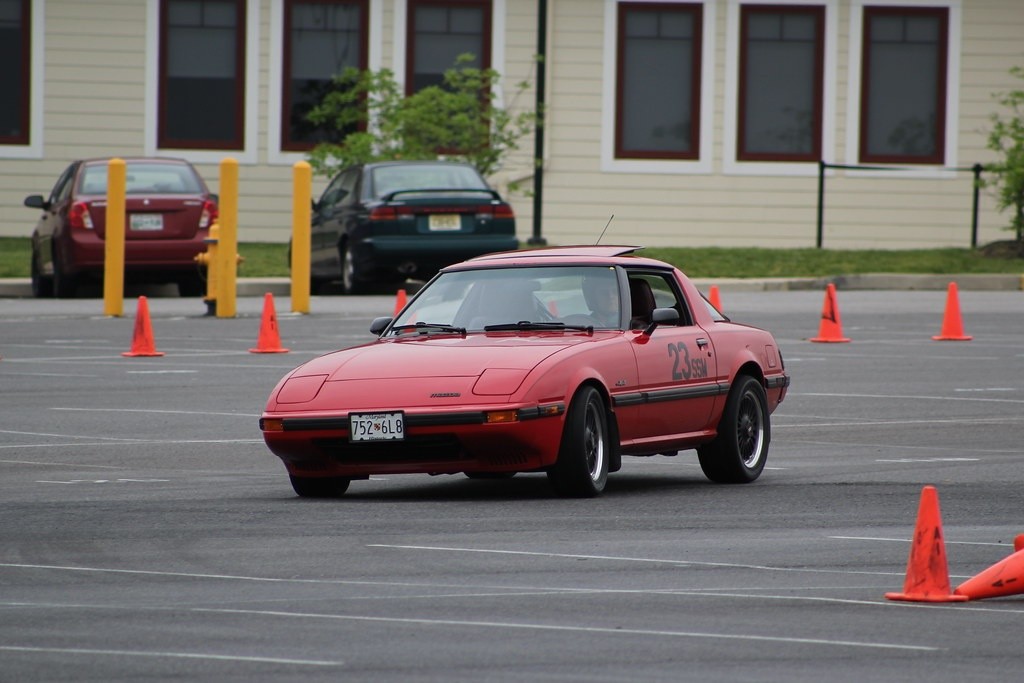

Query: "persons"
left=590, top=274, right=619, bottom=325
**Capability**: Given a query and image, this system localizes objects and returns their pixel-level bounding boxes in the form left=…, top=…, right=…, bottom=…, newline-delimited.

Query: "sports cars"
left=256, top=242, right=791, bottom=500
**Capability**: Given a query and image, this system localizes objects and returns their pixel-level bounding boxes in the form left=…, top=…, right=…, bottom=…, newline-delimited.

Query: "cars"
left=19, top=154, right=220, bottom=297
left=284, top=156, right=519, bottom=297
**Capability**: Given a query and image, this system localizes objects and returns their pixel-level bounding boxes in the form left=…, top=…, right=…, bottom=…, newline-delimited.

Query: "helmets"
left=582, top=269, right=619, bottom=310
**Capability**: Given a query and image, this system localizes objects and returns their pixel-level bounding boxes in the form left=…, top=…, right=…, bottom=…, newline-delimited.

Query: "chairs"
left=468, top=277, right=546, bottom=329
left=627, top=278, right=657, bottom=326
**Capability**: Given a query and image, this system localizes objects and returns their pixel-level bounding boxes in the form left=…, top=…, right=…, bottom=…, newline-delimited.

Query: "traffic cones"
left=881, top=484, right=969, bottom=603
left=545, top=301, right=559, bottom=317
left=954, top=532, right=1024, bottom=601
left=120, top=294, right=165, bottom=356
left=809, top=283, right=855, bottom=343
left=708, top=282, right=722, bottom=314
left=391, top=290, right=416, bottom=332
left=930, top=280, right=973, bottom=341
left=249, top=292, right=290, bottom=353
